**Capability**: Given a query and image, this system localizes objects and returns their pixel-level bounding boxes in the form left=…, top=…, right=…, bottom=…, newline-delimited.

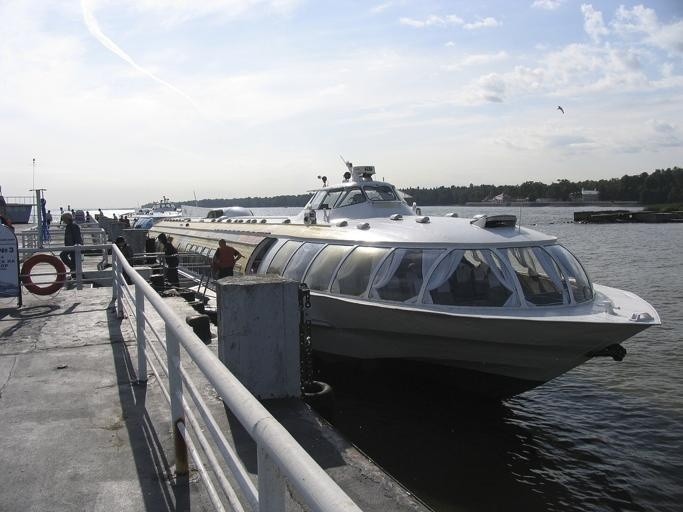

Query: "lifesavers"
left=303, top=380, right=333, bottom=403
left=21, top=253, right=66, bottom=296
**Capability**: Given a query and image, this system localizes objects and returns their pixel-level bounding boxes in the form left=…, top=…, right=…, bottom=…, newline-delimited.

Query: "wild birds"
left=556, top=105, right=564, bottom=114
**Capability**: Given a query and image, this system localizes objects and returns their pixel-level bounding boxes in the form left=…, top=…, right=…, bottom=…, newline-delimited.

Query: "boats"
left=118, top=194, right=184, bottom=229
left=141, top=154, right=663, bottom=409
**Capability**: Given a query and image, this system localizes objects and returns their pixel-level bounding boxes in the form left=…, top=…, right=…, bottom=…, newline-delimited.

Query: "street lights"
left=32, top=157, right=36, bottom=226
left=29, top=187, right=45, bottom=248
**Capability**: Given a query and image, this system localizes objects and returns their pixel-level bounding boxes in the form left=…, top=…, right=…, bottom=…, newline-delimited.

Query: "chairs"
left=338, top=263, right=506, bottom=307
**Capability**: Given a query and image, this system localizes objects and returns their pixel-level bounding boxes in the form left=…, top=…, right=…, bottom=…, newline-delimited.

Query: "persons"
left=59, top=213, right=85, bottom=279
left=212, top=240, right=242, bottom=279
left=99, top=209, right=104, bottom=219
left=114, top=237, right=135, bottom=285
left=156, top=232, right=181, bottom=288
left=86, top=211, right=91, bottom=223
left=46, top=210, right=52, bottom=229
left=111, top=213, right=131, bottom=229
left=58, top=205, right=78, bottom=225
